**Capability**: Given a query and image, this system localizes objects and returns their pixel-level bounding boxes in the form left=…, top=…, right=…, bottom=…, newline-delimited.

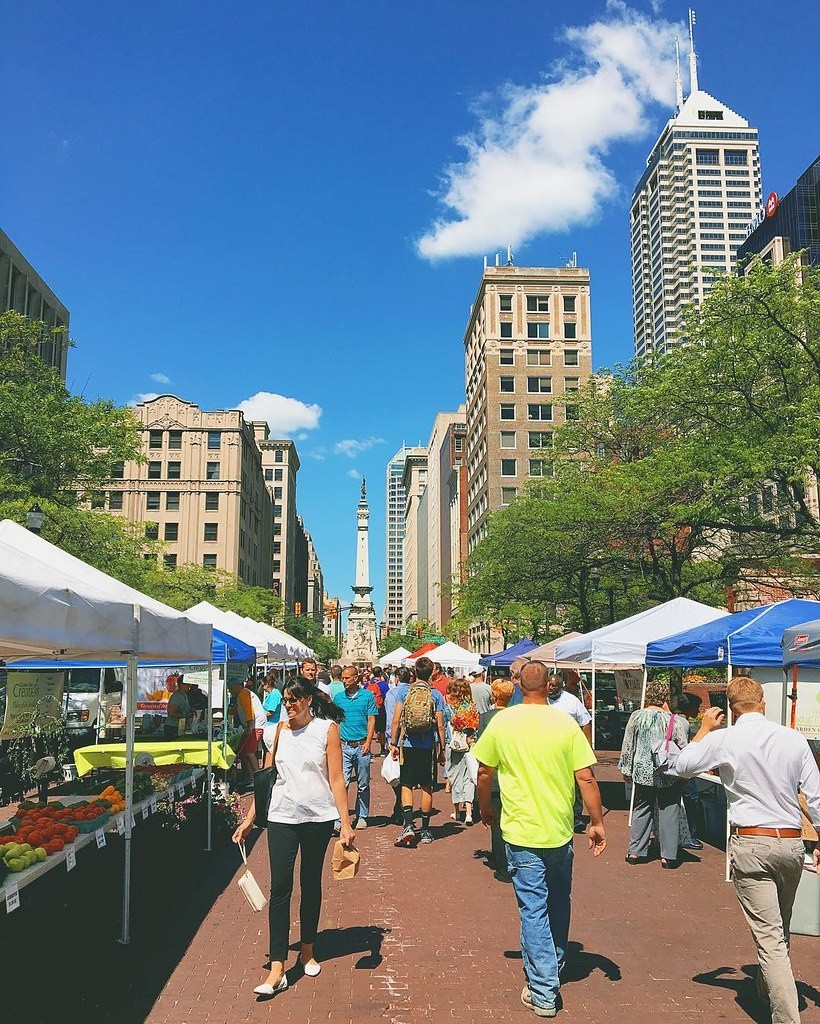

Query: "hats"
left=166, top=674, right=178, bottom=682
left=470, top=665, right=487, bottom=675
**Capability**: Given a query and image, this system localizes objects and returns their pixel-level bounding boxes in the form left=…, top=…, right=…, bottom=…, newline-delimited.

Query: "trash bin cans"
left=210, top=782, right=230, bottom=802
left=63, top=763, right=77, bottom=781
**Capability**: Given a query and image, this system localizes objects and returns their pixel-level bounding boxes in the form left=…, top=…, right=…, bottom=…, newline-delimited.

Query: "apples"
left=0, top=842, right=47, bottom=872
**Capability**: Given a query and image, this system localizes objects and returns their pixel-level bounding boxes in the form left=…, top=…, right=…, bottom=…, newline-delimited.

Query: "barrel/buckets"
left=63, top=764, right=77, bottom=781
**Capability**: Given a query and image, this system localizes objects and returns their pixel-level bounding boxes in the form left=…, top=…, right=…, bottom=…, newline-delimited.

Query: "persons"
left=165, top=655, right=820, bottom=1024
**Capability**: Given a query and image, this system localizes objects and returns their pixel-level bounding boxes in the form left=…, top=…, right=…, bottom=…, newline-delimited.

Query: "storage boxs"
left=61, top=812, right=109, bottom=833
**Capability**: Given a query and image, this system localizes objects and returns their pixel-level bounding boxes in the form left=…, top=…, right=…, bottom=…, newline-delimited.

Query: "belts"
left=340, top=739, right=366, bottom=748
left=730, top=825, right=802, bottom=837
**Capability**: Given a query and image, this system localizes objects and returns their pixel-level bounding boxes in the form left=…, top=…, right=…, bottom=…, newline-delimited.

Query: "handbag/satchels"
left=448, top=730, right=469, bottom=752
left=237, top=839, right=268, bottom=913
left=650, top=713, right=691, bottom=779
left=797, top=790, right=819, bottom=841
left=253, top=721, right=283, bottom=827
left=381, top=751, right=401, bottom=787
left=370, top=731, right=381, bottom=754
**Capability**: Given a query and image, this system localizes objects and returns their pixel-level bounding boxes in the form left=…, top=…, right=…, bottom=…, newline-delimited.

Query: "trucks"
left=61, top=667, right=182, bottom=744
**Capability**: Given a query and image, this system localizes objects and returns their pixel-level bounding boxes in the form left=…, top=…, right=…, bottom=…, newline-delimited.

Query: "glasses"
left=279, top=694, right=308, bottom=705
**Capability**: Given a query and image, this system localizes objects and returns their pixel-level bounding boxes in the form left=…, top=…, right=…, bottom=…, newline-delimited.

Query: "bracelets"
left=440, top=749, right=445, bottom=751
left=390, top=742, right=395, bottom=746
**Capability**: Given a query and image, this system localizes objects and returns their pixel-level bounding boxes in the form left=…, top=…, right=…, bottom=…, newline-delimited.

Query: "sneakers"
left=394, top=826, right=415, bottom=845
left=331, top=824, right=341, bottom=836
left=420, top=833, right=434, bottom=843
left=356, top=818, right=367, bottom=829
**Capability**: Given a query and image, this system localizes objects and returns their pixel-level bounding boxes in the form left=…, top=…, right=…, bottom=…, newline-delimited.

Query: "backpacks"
left=399, top=680, right=436, bottom=734
left=366, top=681, right=383, bottom=708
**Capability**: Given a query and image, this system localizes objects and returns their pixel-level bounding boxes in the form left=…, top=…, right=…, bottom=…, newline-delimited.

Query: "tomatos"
left=0, top=805, right=105, bottom=855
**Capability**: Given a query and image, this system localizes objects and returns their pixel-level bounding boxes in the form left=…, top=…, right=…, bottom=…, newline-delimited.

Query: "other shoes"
left=625, top=854, right=639, bottom=863
left=661, top=858, right=675, bottom=867
left=445, top=787, right=450, bottom=793
left=379, top=749, right=389, bottom=757
left=450, top=814, right=460, bottom=820
left=246, top=782, right=254, bottom=787
left=521, top=985, right=556, bottom=1016
left=690, top=840, right=703, bottom=849
left=253, top=973, right=288, bottom=995
left=574, top=821, right=585, bottom=833
left=465, top=817, right=473, bottom=825
left=299, top=954, right=320, bottom=976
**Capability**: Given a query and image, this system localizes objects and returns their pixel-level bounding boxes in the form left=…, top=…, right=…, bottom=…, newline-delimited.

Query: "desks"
left=0, top=768, right=206, bottom=904
left=73, top=738, right=235, bottom=776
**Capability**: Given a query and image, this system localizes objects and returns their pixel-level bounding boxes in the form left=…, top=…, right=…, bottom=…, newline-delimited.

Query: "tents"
left=0, top=520, right=319, bottom=949
left=378, top=595, right=820, bottom=883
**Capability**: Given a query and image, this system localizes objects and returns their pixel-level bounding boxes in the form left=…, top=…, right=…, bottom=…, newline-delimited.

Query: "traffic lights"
left=416, top=627, right=422, bottom=639
left=386, top=625, right=391, bottom=637
